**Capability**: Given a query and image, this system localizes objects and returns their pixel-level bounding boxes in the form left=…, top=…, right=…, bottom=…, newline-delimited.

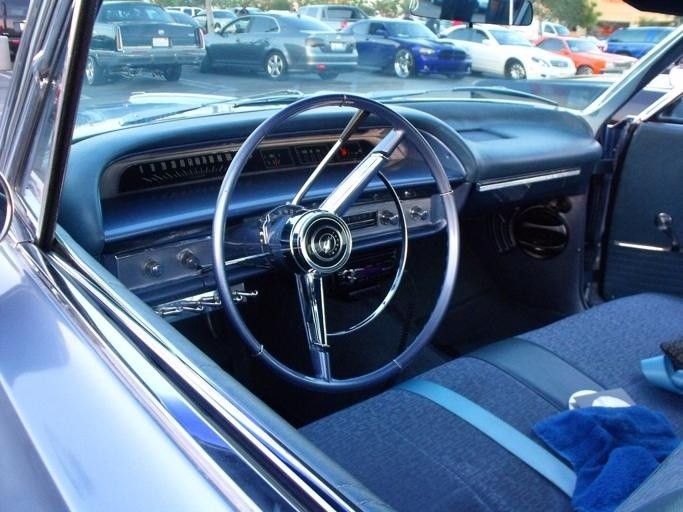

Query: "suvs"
left=0, top=0, right=33, bottom=62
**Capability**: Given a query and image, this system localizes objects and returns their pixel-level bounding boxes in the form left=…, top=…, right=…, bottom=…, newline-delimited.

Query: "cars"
left=193, top=13, right=358, bottom=82
left=86, top=0, right=206, bottom=86
left=0, top=0, right=683, bottom=512
left=531, top=34, right=641, bottom=76
left=338, top=19, right=473, bottom=83
left=435, top=23, right=577, bottom=82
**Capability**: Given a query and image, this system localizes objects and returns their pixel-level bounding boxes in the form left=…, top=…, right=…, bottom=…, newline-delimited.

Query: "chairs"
left=296, top=292, right=682, bottom=512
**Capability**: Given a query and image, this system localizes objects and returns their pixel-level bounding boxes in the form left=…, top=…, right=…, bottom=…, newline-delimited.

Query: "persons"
left=233, top=2, right=250, bottom=18
left=425, top=17, right=441, bottom=35
left=403, top=8, right=413, bottom=21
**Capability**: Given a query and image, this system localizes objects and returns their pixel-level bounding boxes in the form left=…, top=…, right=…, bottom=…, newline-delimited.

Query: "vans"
left=602, top=24, right=677, bottom=61
